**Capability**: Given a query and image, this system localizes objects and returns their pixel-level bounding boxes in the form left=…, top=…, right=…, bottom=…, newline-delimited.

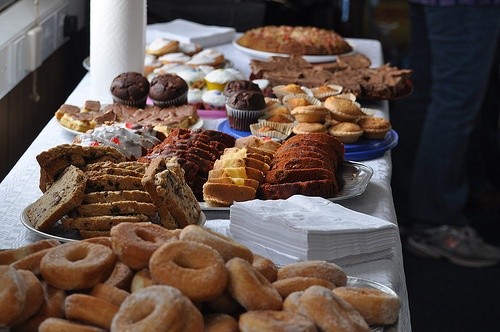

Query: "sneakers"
left=408, top=220, right=500, bottom=267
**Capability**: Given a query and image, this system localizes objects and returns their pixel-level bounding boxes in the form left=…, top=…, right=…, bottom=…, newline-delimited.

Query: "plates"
left=217, top=119, right=398, bottom=160
left=145, top=96, right=227, bottom=117
left=195, top=161, right=373, bottom=210
left=345, top=276, right=399, bottom=332
left=20, top=203, right=206, bottom=242
left=232, top=37, right=356, bottom=63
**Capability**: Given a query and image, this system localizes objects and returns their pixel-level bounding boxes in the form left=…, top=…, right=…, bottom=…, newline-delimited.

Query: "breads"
left=237, top=26, right=353, bottom=56
left=24, top=144, right=201, bottom=238
left=57, top=39, right=412, bottom=205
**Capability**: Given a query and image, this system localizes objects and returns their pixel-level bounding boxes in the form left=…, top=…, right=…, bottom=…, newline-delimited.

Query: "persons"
left=390, top=0, right=500, bottom=267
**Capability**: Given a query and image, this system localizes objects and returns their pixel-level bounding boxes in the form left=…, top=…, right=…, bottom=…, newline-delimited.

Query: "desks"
left=0, top=32, right=412, bottom=332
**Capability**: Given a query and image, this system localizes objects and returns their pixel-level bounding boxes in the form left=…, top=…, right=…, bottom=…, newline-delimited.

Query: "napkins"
left=148, top=19, right=235, bottom=47
left=225, top=195, right=399, bottom=268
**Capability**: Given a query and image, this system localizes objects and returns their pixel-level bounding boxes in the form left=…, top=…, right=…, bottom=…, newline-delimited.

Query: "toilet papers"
left=89, top=0, right=147, bottom=105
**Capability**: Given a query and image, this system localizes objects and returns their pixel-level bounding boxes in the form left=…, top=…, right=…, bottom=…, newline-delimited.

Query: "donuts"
left=0, top=222, right=401, bottom=332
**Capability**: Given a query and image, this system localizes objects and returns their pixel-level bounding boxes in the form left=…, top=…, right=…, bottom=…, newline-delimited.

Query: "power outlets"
left=11, top=2, right=71, bottom=86
